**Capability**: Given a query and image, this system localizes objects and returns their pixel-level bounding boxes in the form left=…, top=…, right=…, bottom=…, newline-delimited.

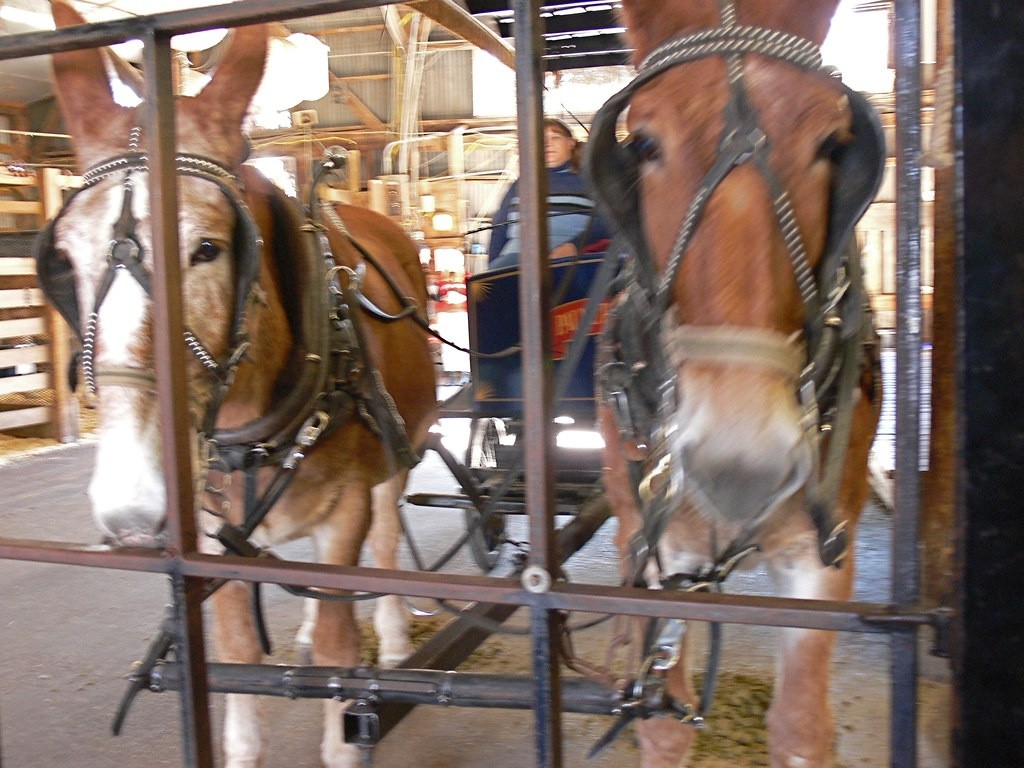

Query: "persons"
left=486, top=116, right=613, bottom=271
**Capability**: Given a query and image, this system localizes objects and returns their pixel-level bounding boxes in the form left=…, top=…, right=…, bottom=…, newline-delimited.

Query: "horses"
left=599, top=0, right=883, bottom=768
left=51, top=2, right=440, bottom=767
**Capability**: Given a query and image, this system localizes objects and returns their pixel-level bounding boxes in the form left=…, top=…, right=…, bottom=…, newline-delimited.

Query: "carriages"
left=34, top=0, right=892, bottom=767
